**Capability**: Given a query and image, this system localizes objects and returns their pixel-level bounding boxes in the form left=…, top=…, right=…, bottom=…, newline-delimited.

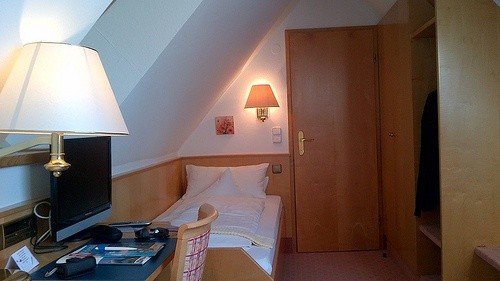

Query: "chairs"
left=170, top=203, right=219, bottom=281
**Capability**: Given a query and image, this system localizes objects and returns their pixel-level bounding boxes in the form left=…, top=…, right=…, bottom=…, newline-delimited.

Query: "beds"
left=152, top=194, right=285, bottom=281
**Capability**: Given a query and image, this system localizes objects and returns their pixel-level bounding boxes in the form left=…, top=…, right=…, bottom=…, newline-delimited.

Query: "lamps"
left=0, top=42, right=130, bottom=177
left=244, top=85, right=280, bottom=122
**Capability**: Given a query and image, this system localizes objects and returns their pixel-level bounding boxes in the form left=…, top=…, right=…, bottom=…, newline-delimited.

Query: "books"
left=56, top=243, right=164, bottom=265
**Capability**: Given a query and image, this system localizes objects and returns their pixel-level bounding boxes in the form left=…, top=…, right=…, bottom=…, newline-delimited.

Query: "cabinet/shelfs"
left=377, top=0, right=500, bottom=281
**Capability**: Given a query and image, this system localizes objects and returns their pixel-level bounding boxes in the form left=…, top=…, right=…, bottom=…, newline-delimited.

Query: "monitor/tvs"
left=50, top=136, right=114, bottom=243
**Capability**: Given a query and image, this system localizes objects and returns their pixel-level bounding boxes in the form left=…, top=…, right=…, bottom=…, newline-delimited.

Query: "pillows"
left=182, top=163, right=269, bottom=198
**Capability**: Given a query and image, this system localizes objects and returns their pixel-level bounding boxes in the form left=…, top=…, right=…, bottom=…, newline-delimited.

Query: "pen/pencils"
left=95, top=247, right=138, bottom=251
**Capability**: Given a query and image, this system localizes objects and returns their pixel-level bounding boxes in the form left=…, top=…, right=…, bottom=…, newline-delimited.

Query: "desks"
left=0, top=222, right=177, bottom=281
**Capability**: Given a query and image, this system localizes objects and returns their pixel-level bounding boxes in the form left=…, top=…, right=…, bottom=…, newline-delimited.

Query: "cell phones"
left=109, top=221, right=152, bottom=226
